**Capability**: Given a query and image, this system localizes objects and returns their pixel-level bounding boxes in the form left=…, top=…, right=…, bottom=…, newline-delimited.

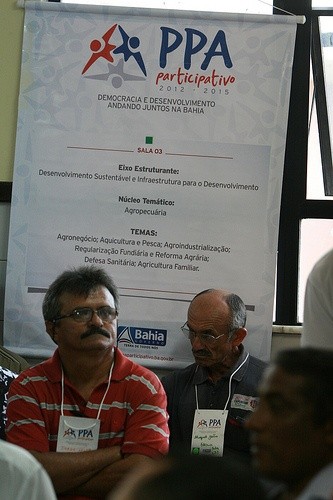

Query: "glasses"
left=53, top=305, right=119, bottom=323
left=248, top=398, right=321, bottom=422
left=179, top=321, right=231, bottom=344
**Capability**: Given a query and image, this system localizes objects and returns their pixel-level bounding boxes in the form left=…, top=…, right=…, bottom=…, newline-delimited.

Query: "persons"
left=0, top=248, right=333, bottom=500
